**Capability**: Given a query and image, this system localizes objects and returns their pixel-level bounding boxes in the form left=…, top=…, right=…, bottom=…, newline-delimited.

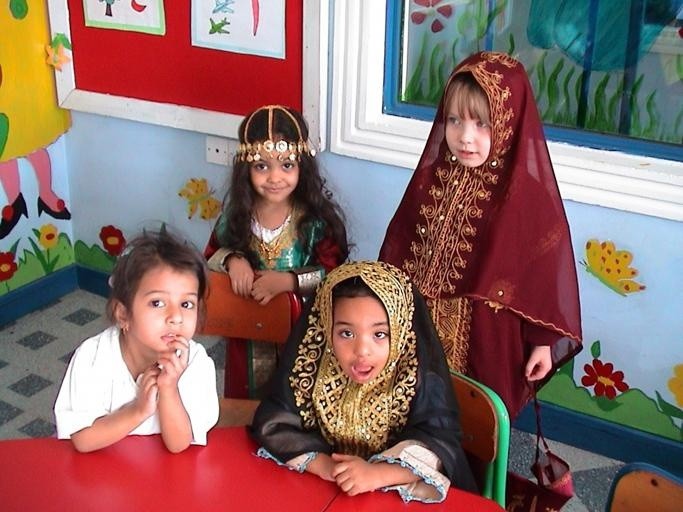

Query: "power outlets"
left=206, top=137, right=230, bottom=167
left=231, top=141, right=242, bottom=163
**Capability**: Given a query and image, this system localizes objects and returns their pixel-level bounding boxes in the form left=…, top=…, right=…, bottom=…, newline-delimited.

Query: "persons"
left=249, top=258, right=483, bottom=507
left=54, top=220, right=220, bottom=454
left=376, top=47, right=584, bottom=423
left=199, top=102, right=350, bottom=402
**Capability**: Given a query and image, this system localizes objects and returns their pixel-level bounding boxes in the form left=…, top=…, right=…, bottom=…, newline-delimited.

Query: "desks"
left=0, top=426, right=505, bottom=512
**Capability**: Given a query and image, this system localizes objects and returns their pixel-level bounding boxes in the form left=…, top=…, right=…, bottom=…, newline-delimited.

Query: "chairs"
left=442, top=368, right=510, bottom=512
left=196, top=269, right=305, bottom=427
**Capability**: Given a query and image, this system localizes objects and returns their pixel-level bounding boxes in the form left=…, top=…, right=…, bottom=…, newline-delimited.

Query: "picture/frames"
left=330, top=0, right=683, bottom=222
left=48, top=0, right=329, bottom=153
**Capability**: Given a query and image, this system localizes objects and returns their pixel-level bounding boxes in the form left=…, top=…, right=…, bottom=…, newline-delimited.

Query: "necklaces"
left=253, top=196, right=295, bottom=261
